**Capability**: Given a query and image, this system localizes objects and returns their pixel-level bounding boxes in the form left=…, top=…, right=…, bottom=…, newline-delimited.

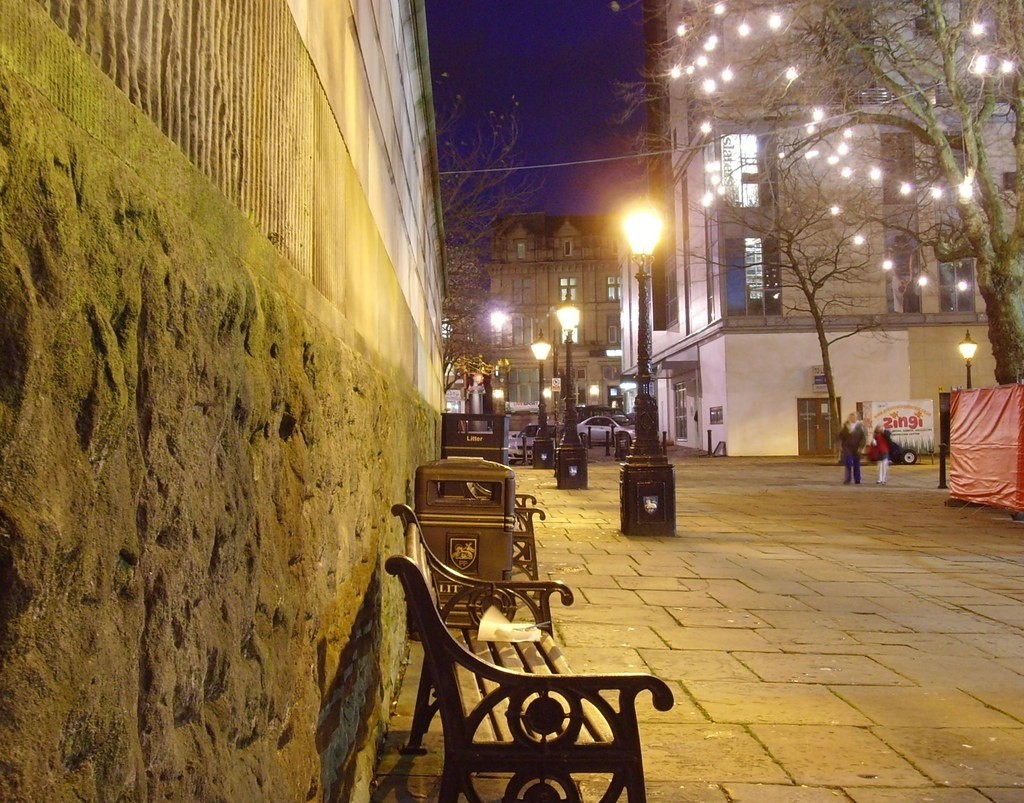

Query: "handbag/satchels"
left=837, top=450, right=844, bottom=463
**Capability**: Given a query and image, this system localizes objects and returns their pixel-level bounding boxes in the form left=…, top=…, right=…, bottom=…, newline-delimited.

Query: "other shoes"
left=844, top=480, right=850, bottom=484
left=855, top=480, right=860, bottom=484
left=876, top=480, right=887, bottom=485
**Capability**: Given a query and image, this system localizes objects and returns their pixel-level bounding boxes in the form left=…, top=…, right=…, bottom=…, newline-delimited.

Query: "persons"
left=870, top=425, right=892, bottom=485
left=839, top=413, right=866, bottom=484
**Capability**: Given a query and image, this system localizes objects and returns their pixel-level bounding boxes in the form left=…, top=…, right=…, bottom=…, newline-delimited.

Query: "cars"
left=577, top=415, right=635, bottom=447
left=512, top=422, right=564, bottom=465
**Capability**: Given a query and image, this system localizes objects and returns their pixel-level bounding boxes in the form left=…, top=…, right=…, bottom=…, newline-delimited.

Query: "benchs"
left=384, top=501, right=674, bottom=803
left=459, top=481, right=545, bottom=581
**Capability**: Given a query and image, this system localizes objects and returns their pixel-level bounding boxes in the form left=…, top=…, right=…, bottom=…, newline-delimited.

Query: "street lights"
left=555, top=306, right=590, bottom=489
left=958, top=330, right=978, bottom=389
left=532, top=328, right=557, bottom=469
left=619, top=203, right=677, bottom=536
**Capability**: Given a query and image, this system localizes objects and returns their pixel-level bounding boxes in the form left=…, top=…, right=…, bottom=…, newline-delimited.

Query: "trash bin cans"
left=414, top=458, right=517, bottom=627
left=440, top=412, right=511, bottom=495
